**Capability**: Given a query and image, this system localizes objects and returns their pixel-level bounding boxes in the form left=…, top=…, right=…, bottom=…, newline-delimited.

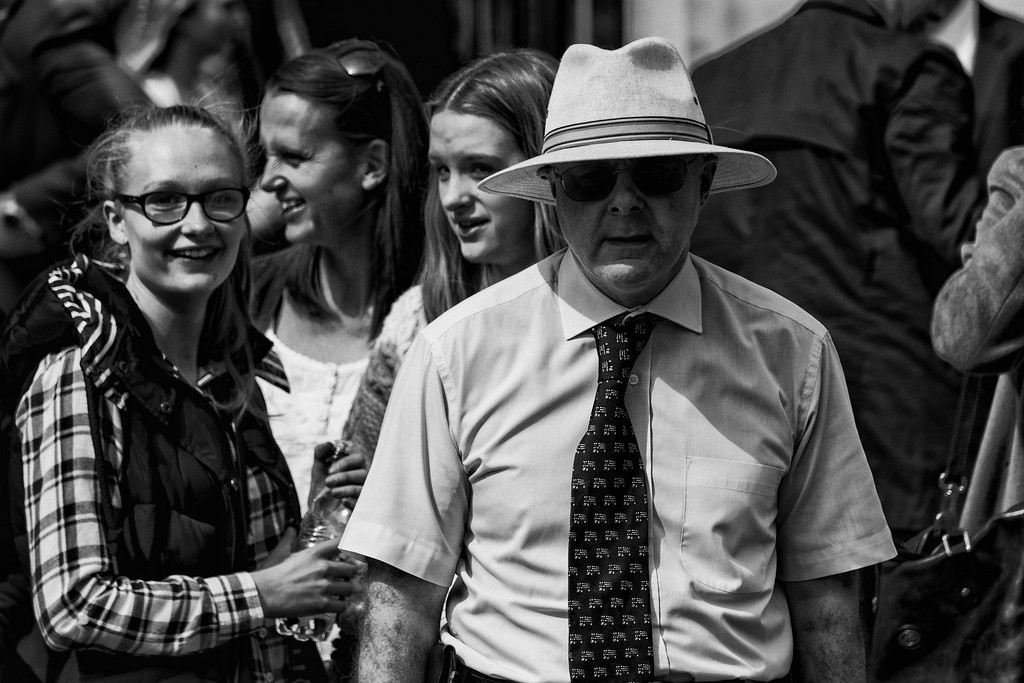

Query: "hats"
left=477, top=38, right=779, bottom=202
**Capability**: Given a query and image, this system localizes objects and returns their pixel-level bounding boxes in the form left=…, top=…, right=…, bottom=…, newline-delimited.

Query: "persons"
left=0, top=0, right=1024, bottom=683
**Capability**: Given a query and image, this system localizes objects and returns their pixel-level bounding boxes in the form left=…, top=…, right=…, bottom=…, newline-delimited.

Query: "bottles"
left=274, top=485, right=351, bottom=643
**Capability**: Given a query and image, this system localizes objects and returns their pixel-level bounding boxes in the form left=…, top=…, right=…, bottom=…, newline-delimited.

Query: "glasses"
left=327, top=40, right=387, bottom=107
left=115, top=185, right=247, bottom=225
left=556, top=154, right=686, bottom=203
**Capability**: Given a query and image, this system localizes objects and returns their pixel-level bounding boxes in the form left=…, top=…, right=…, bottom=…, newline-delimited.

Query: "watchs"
left=2, top=200, right=31, bottom=245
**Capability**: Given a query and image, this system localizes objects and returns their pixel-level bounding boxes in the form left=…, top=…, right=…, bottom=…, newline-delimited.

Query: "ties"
left=567, top=319, right=656, bottom=683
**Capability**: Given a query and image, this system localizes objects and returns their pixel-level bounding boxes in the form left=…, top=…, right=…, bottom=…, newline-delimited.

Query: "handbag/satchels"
left=866, top=358, right=1024, bottom=683
